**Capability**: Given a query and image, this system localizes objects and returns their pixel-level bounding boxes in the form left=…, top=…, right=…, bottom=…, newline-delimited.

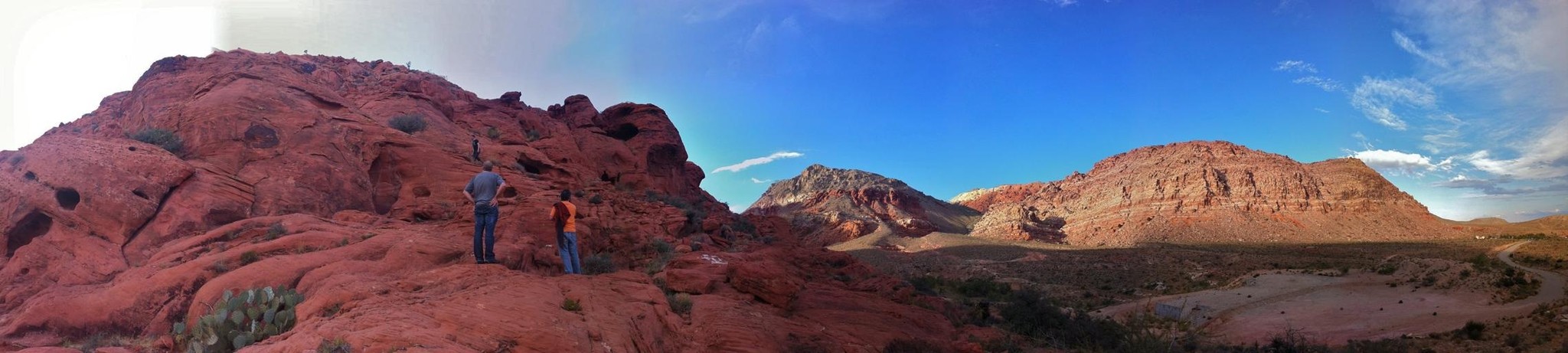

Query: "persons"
left=549, top=189, right=583, bottom=273
left=462, top=158, right=506, bottom=264
left=471, top=132, right=483, bottom=164
